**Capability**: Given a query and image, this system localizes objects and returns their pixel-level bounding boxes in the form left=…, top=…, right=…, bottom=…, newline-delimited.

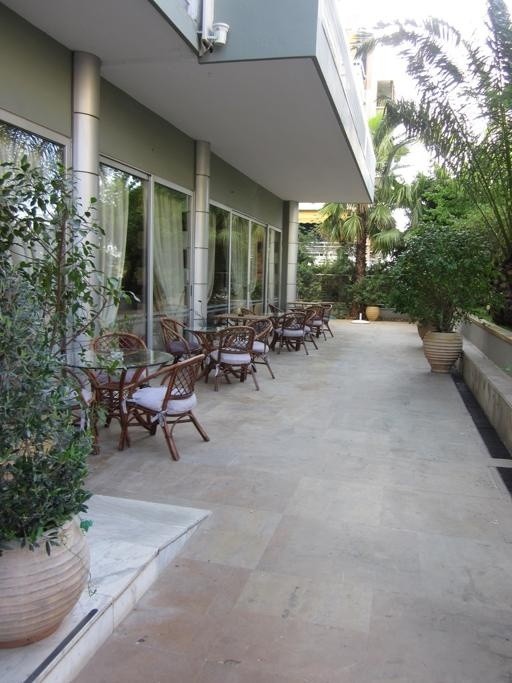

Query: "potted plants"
left=383, top=219, right=503, bottom=374
left=0, top=152, right=141, bottom=650
left=346, top=272, right=389, bottom=321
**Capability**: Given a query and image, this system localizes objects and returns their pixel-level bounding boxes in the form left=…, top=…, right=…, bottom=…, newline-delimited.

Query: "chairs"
left=31, top=369, right=100, bottom=455
left=93, top=303, right=335, bottom=460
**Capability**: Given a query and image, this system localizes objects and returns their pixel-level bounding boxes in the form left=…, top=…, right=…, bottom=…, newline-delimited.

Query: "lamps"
left=202, top=23, right=230, bottom=55
